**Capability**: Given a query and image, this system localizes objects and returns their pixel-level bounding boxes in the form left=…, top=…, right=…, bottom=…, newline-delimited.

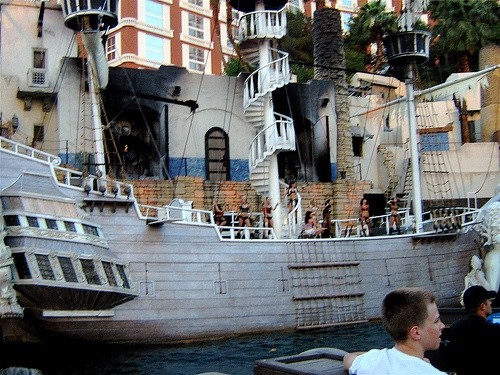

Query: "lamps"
left=11, top=113, right=19, bottom=134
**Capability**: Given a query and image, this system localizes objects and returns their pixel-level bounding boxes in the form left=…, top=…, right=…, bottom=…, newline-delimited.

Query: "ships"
left=2, top=2, right=500, bottom=352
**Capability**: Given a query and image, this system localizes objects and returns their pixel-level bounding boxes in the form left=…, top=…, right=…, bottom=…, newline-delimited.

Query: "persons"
left=0, top=115, right=402, bottom=242
left=450, top=286, right=500, bottom=375
left=344, top=286, right=451, bottom=375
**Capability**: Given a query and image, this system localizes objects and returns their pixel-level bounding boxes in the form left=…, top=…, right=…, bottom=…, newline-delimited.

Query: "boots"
left=397, top=227, right=401, bottom=235
left=389, top=228, right=393, bottom=235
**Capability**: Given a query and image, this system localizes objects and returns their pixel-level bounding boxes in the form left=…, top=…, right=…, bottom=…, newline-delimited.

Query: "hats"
left=463, top=285, right=497, bottom=311
